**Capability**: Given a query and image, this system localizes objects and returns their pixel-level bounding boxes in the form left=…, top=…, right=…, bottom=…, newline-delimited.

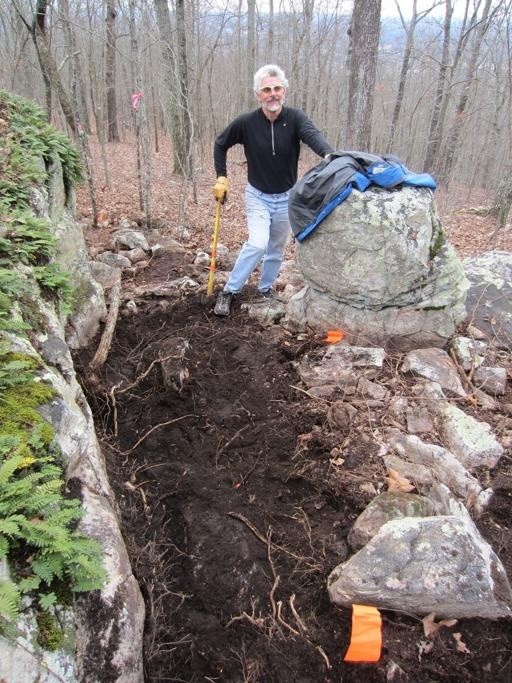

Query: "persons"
left=212, top=60, right=339, bottom=318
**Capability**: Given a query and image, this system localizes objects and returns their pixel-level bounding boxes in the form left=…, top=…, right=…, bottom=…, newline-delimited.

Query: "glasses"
left=256, top=85, right=283, bottom=92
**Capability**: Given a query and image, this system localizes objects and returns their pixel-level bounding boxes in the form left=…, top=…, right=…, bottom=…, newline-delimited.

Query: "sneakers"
left=213, top=290, right=233, bottom=315
left=258, top=287, right=278, bottom=299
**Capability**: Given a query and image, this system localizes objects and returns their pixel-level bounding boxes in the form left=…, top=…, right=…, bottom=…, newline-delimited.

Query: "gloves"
left=213, top=176, right=229, bottom=205
